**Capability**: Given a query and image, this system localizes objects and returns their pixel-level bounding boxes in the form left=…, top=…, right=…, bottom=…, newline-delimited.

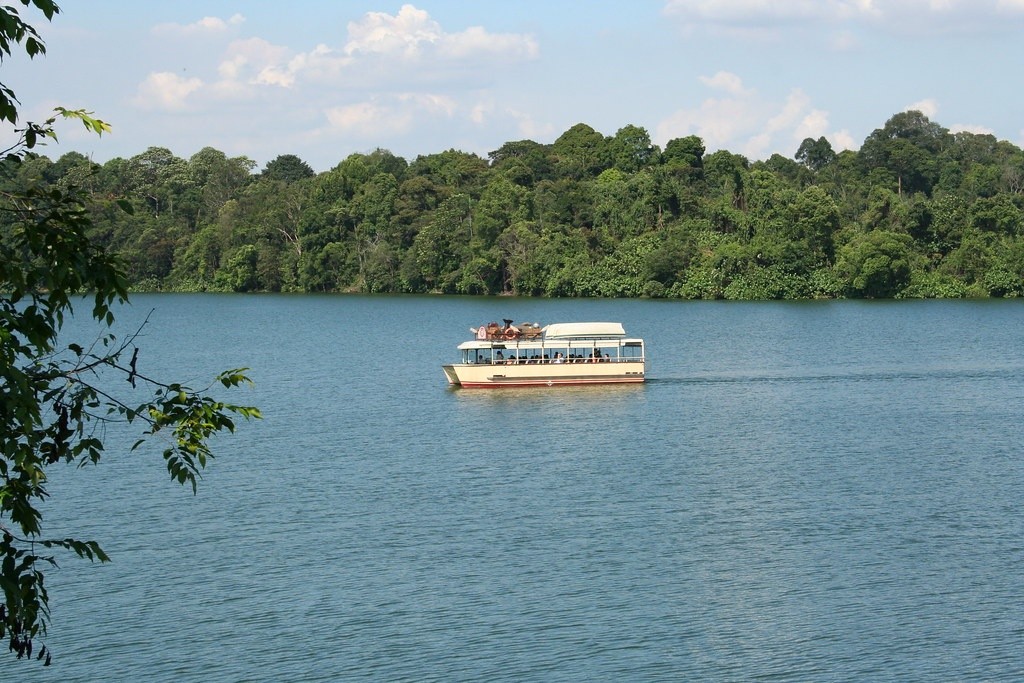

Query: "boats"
left=440, top=318, right=647, bottom=387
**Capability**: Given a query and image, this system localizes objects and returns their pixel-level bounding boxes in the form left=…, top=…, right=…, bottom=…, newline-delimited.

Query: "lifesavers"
left=504, top=328, right=516, bottom=340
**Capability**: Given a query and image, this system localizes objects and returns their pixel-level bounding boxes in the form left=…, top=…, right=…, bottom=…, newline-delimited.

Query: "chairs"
left=503, top=359, right=611, bottom=364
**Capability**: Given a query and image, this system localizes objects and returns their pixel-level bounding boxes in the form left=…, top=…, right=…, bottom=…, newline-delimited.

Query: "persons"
left=604, top=354, right=610, bottom=362
left=553, top=353, right=565, bottom=363
left=505, top=348, right=604, bottom=364
left=496, top=351, right=504, bottom=364
left=478, top=355, right=486, bottom=364
